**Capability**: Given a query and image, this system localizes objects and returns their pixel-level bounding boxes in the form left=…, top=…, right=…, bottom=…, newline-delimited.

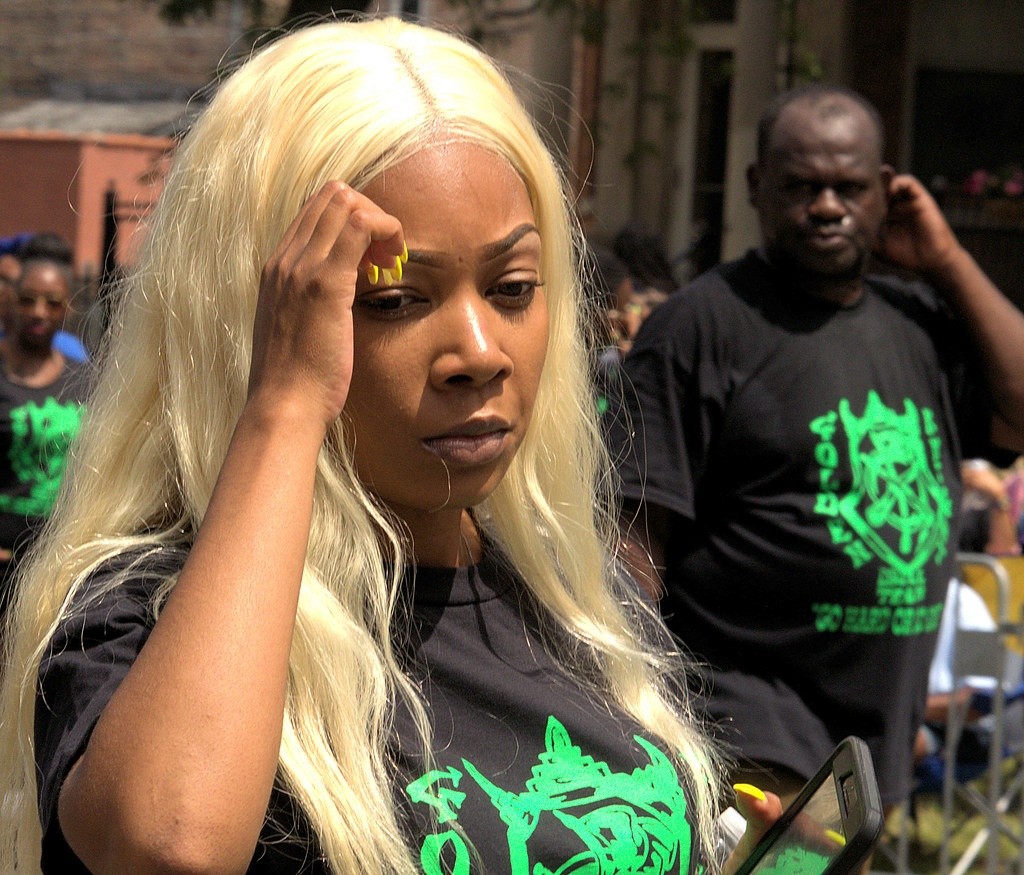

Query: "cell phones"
left=733, top=735, right=885, bottom=875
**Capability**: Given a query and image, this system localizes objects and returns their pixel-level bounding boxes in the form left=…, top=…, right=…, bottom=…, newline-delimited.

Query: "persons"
left=597, top=79, right=1024, bottom=874
left=0, top=17, right=785, bottom=874
left=0, top=231, right=1024, bottom=802
